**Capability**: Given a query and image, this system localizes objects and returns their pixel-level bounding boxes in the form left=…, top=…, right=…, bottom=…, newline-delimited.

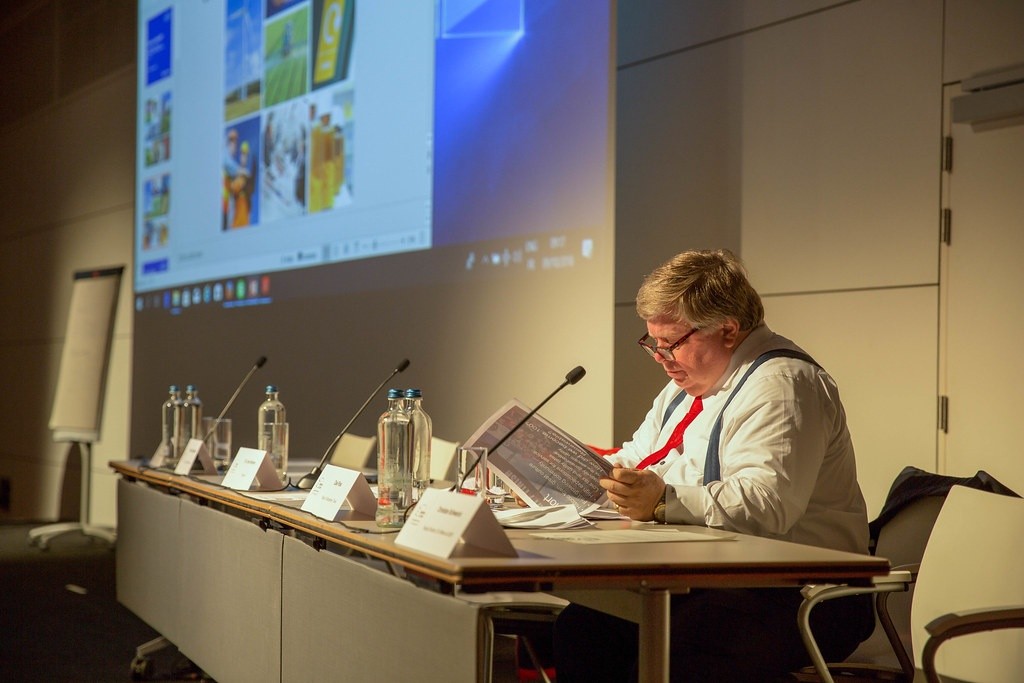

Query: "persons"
left=512, top=249, right=877, bottom=683
left=221, top=129, right=255, bottom=234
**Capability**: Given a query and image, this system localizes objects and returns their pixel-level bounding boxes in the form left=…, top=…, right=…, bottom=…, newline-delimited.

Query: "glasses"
left=638, top=328, right=699, bottom=362
left=280, top=473, right=318, bottom=489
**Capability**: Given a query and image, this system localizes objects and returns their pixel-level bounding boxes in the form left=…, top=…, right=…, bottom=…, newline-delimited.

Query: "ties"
left=636, top=394, right=703, bottom=472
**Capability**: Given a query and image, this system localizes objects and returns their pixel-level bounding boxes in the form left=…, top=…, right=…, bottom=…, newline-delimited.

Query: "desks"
left=108, top=461, right=891, bottom=683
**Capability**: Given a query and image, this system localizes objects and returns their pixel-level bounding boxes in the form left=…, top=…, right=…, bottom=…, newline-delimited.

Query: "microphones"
left=455, top=365, right=586, bottom=492
left=297, top=358, right=411, bottom=488
left=191, top=356, right=269, bottom=470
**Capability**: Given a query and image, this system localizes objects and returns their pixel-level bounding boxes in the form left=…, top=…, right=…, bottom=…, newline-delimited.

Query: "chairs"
left=791, top=466, right=1024, bottom=683
left=415, top=435, right=459, bottom=481
left=457, top=585, right=570, bottom=683
left=330, top=433, right=378, bottom=468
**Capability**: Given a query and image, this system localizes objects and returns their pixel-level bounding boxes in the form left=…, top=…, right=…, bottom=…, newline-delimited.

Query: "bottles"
left=257, top=385, right=287, bottom=486
left=176, top=385, right=202, bottom=465
left=374, top=389, right=433, bottom=529
left=162, top=385, right=183, bottom=468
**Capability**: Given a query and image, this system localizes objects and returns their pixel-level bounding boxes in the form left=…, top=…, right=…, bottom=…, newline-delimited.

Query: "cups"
left=200, top=416, right=213, bottom=458
left=487, top=467, right=505, bottom=509
left=456, top=447, right=487, bottom=499
left=212, top=419, right=232, bottom=471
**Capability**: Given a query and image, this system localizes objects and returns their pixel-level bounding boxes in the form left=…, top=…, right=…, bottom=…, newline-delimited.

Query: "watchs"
left=652, top=487, right=669, bottom=522
